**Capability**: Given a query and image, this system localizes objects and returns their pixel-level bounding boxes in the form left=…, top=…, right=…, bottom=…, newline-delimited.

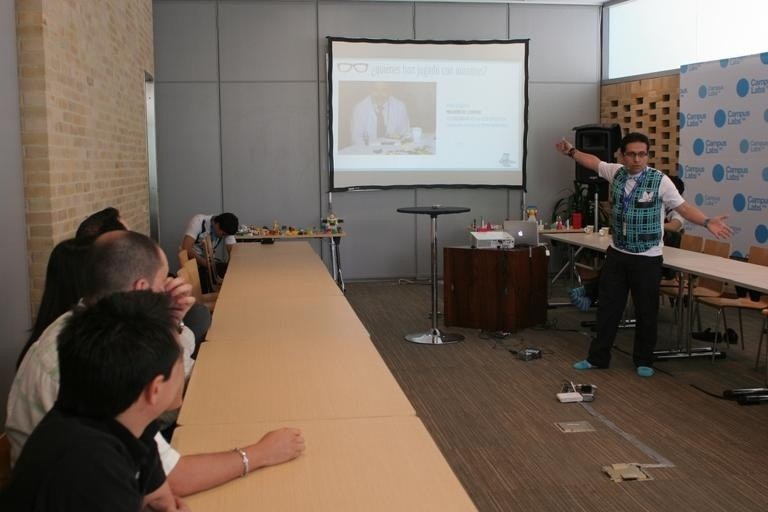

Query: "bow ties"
left=624, top=175, right=640, bottom=184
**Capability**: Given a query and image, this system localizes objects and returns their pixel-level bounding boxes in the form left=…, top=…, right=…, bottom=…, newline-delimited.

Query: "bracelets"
left=234, top=447, right=249, bottom=478
left=703, top=217, right=711, bottom=228
left=568, top=147, right=577, bottom=159
left=177, top=322, right=184, bottom=334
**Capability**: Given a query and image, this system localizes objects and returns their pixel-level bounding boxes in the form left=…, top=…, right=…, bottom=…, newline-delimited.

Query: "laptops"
left=503, top=219, right=541, bottom=246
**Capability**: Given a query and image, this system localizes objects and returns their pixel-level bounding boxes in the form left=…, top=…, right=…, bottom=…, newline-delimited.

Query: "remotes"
left=432, top=204, right=441, bottom=208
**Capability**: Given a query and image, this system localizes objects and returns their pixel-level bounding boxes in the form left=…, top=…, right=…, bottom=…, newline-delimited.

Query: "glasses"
left=623, top=150, right=648, bottom=158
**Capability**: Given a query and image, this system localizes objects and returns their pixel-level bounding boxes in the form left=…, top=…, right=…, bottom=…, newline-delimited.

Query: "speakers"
left=573, top=123, right=622, bottom=185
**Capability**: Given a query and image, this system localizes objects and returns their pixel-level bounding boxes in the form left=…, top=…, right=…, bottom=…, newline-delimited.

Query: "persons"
left=1, top=288, right=191, bottom=512
left=6, top=231, right=304, bottom=497
left=556, top=132, right=733, bottom=378
left=75, top=206, right=211, bottom=432
left=567, top=175, right=685, bottom=313
left=350, top=82, right=413, bottom=147
left=180, top=213, right=239, bottom=293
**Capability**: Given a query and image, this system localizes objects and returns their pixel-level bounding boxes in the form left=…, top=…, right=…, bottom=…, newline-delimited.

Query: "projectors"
left=469, top=230, right=514, bottom=249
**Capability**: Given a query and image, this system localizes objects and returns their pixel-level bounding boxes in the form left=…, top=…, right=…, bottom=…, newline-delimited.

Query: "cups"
left=362, top=126, right=423, bottom=153
left=584, top=225, right=610, bottom=235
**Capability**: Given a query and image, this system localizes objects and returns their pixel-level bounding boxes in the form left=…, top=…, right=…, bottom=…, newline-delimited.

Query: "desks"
left=441, top=246, right=551, bottom=335
left=396, top=203, right=472, bottom=348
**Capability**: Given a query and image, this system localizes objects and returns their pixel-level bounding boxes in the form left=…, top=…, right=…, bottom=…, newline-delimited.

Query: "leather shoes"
left=690, top=325, right=723, bottom=343
left=721, top=327, right=740, bottom=344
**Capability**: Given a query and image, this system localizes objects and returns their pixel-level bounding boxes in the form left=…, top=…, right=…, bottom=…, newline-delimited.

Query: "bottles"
left=260, top=211, right=344, bottom=238
left=526, top=204, right=581, bottom=231
left=472, top=215, right=503, bottom=231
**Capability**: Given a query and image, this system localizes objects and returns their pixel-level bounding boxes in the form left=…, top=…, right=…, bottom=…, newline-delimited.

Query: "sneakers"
left=636, top=365, right=657, bottom=378
left=572, top=358, right=610, bottom=370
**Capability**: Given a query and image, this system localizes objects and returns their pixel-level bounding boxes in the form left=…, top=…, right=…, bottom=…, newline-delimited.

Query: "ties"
left=375, top=106, right=388, bottom=138
